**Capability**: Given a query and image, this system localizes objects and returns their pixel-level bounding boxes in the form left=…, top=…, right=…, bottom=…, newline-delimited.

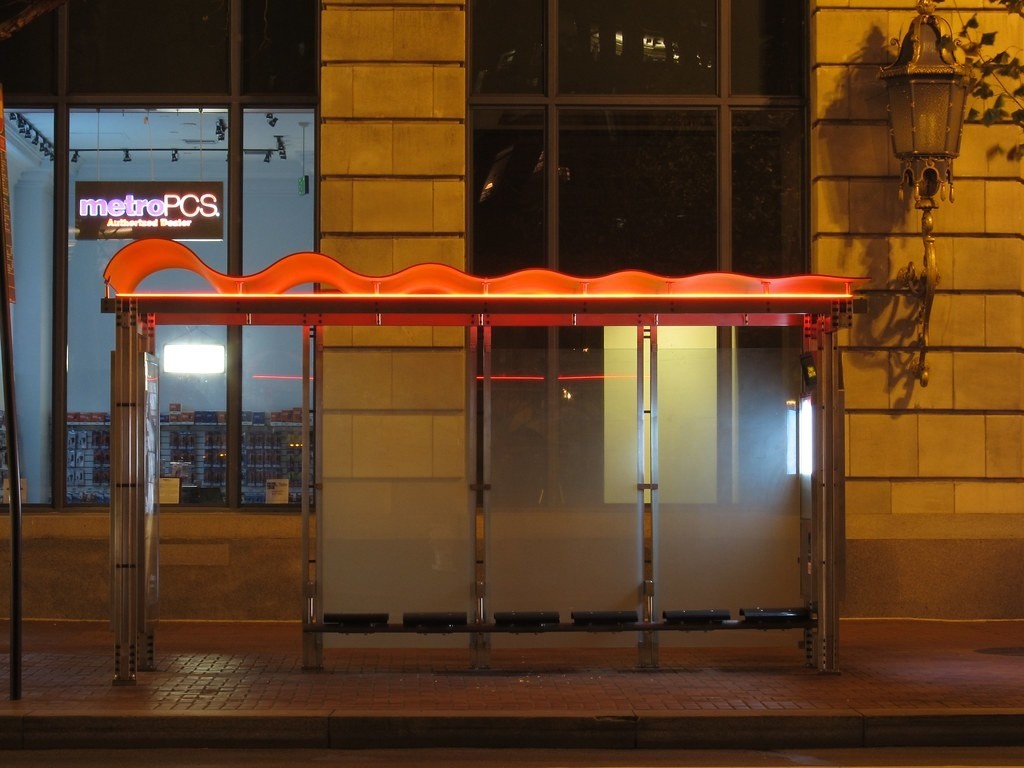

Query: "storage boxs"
left=67, top=403, right=303, bottom=422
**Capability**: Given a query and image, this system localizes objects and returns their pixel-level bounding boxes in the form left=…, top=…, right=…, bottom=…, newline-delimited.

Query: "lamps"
left=71, top=151, right=80, bottom=162
left=276, top=136, right=287, bottom=160
left=264, top=151, right=272, bottom=163
left=880, top=1, right=978, bottom=388
left=172, top=150, right=178, bottom=161
left=266, top=112, right=278, bottom=127
left=10, top=112, right=54, bottom=161
left=123, top=151, right=132, bottom=161
left=216, top=119, right=226, bottom=140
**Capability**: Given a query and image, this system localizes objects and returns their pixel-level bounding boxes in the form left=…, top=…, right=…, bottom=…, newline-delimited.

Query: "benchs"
left=305, top=609, right=810, bottom=633
